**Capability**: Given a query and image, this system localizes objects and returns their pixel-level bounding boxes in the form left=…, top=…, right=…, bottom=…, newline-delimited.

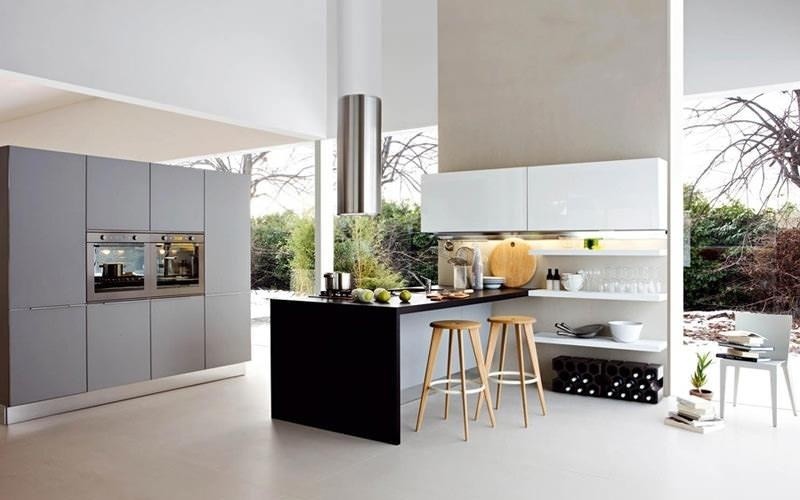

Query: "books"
left=663, top=394, right=725, bottom=434
left=716, top=328, right=776, bottom=364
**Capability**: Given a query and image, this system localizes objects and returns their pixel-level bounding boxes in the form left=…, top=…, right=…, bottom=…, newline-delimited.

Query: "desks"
left=267, top=286, right=534, bottom=446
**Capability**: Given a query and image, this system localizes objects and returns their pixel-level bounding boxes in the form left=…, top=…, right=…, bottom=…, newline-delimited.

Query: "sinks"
left=389, top=288, right=444, bottom=292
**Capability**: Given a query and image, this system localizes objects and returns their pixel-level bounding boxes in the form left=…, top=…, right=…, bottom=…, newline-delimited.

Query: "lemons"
left=374, top=287, right=391, bottom=302
left=359, top=289, right=373, bottom=302
left=399, top=290, right=411, bottom=301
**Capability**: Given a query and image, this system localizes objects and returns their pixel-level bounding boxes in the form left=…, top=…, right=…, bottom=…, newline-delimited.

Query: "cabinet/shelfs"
left=87, top=299, right=151, bottom=392
left=88, top=155, right=150, bottom=232
left=150, top=162, right=204, bottom=233
left=551, top=355, right=664, bottom=404
left=529, top=156, right=669, bottom=234
left=0, top=145, right=87, bottom=409
left=526, top=248, right=669, bottom=352
left=151, top=294, right=204, bottom=378
left=420, top=166, right=528, bottom=235
left=205, top=169, right=252, bottom=371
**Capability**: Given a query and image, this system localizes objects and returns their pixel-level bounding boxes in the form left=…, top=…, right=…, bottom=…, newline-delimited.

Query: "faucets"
left=406, top=271, right=425, bottom=290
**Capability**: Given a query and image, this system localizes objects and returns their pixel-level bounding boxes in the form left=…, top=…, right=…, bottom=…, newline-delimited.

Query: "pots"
left=554, top=319, right=604, bottom=339
left=324, top=271, right=351, bottom=292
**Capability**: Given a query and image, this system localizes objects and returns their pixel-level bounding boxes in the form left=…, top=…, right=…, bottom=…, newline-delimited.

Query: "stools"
left=413, top=318, right=497, bottom=443
left=473, top=314, right=548, bottom=430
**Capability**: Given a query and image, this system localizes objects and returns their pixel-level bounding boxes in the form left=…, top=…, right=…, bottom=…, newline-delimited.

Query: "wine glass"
left=579, top=266, right=661, bottom=295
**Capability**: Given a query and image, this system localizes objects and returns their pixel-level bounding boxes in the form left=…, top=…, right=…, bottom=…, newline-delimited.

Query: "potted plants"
left=689, top=349, right=715, bottom=400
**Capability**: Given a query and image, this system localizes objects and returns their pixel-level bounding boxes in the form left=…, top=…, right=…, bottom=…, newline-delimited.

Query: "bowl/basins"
left=560, top=272, right=585, bottom=292
left=609, top=319, right=643, bottom=344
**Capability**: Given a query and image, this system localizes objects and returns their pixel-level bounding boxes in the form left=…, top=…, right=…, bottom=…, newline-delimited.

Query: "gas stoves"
left=308, top=289, right=397, bottom=300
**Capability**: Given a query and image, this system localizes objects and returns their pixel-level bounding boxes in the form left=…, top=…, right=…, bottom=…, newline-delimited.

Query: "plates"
left=483, top=276, right=506, bottom=291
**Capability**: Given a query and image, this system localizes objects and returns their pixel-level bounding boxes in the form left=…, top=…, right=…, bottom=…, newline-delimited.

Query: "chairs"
left=717, top=309, right=799, bottom=430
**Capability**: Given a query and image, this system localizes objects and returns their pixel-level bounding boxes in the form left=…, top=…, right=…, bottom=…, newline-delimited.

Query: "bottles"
left=472, top=249, right=485, bottom=290
left=425, top=278, right=433, bottom=296
left=563, top=370, right=655, bottom=405
left=546, top=268, right=560, bottom=290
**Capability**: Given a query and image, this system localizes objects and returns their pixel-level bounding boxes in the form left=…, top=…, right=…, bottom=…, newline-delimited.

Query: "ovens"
left=88, top=230, right=204, bottom=304
